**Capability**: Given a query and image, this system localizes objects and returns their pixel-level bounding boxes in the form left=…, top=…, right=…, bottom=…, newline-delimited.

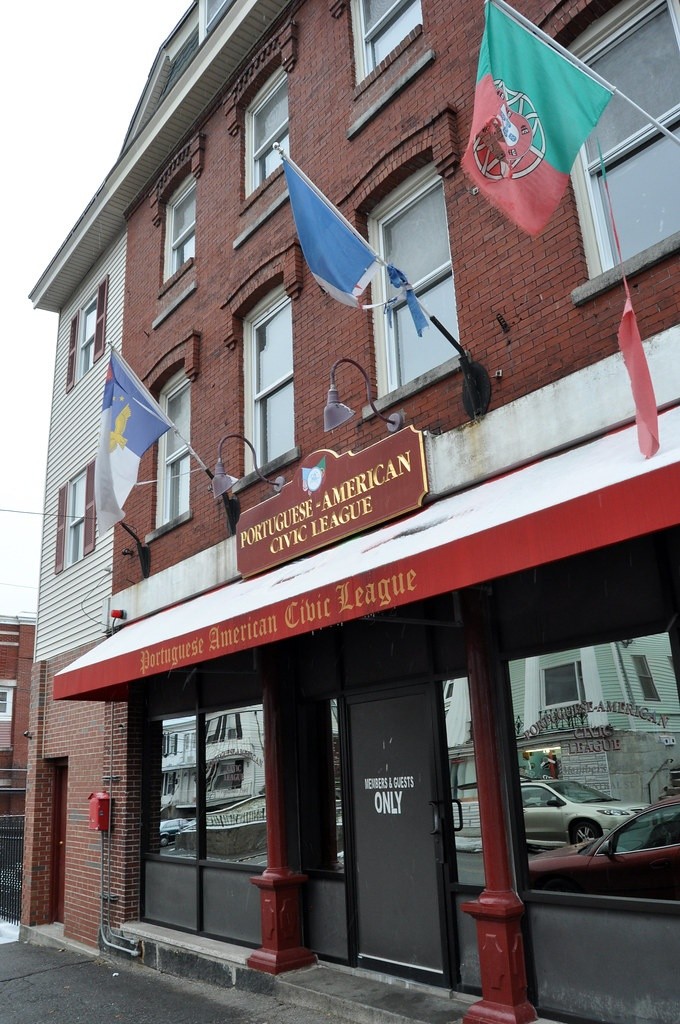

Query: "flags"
left=282, top=160, right=388, bottom=310
left=462, top=0, right=613, bottom=237
left=96, top=353, right=171, bottom=538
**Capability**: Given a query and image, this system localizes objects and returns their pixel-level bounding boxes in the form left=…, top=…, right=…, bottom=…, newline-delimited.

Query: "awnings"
left=52, top=404, right=679, bottom=703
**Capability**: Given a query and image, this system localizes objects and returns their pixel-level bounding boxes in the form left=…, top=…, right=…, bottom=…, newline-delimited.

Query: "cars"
left=519, top=779, right=655, bottom=853
left=526, top=794, right=680, bottom=900
left=158, top=817, right=193, bottom=847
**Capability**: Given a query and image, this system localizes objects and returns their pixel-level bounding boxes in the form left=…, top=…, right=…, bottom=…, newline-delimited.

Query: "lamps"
left=212, top=433, right=286, bottom=499
left=323, top=358, right=403, bottom=434
